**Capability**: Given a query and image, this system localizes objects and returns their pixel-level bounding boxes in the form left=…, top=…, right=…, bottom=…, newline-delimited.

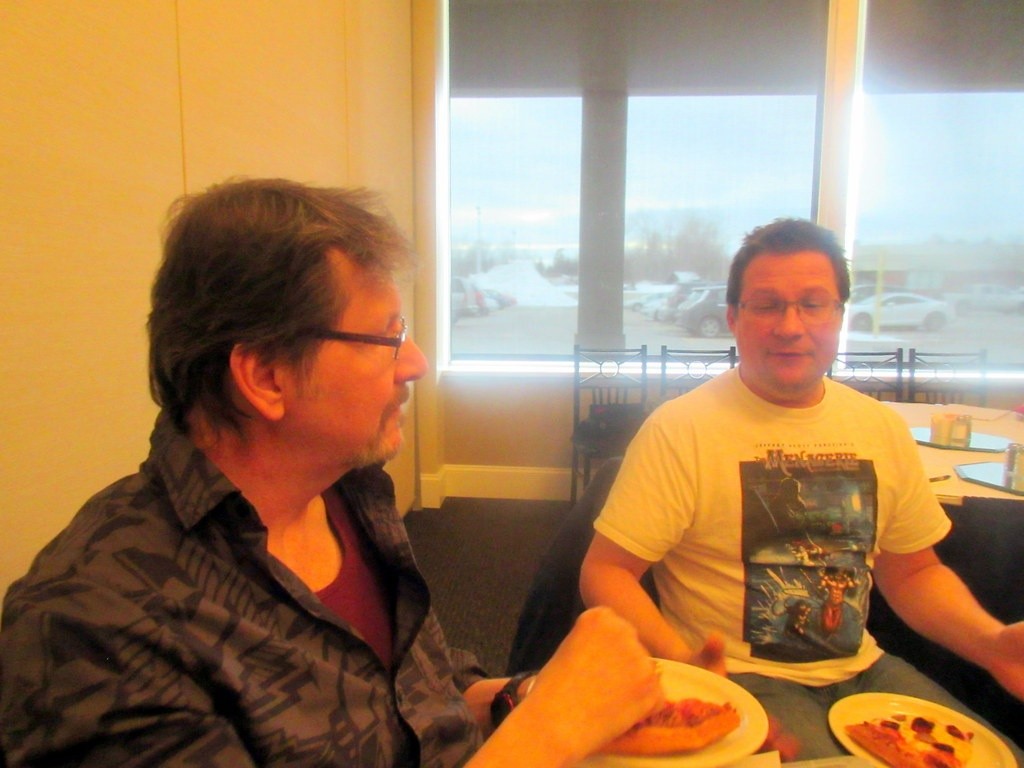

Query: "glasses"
left=295, top=319, right=410, bottom=361
left=735, top=296, right=846, bottom=322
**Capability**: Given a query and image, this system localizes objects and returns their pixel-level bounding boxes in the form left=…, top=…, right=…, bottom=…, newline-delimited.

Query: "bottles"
left=1003, top=443, right=1024, bottom=494
left=928, top=404, right=972, bottom=451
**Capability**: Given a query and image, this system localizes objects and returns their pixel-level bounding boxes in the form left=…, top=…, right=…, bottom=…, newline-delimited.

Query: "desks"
left=880, top=401, right=1024, bottom=506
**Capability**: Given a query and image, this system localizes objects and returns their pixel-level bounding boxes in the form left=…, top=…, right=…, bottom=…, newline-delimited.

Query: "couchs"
left=505, top=456, right=1024, bottom=752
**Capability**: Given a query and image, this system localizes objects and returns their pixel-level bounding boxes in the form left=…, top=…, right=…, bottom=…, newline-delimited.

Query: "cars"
left=640, top=298, right=671, bottom=319
left=952, top=284, right=1024, bottom=318
left=847, top=293, right=951, bottom=333
left=624, top=290, right=673, bottom=311
left=465, top=288, right=517, bottom=315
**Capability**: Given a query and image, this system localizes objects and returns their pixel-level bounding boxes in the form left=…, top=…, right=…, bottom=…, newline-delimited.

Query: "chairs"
left=659, top=343, right=736, bottom=408
left=827, top=347, right=903, bottom=402
left=568, top=341, right=647, bottom=506
left=907, top=347, right=989, bottom=406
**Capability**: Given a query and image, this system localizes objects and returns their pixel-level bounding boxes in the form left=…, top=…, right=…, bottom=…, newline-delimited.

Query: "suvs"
left=668, top=281, right=729, bottom=323
left=674, top=286, right=732, bottom=337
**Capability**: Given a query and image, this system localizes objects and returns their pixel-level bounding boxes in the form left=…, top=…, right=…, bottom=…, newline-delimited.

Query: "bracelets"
left=524, top=677, right=536, bottom=698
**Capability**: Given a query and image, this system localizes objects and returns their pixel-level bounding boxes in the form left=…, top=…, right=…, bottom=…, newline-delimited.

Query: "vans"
left=450, top=276, right=465, bottom=324
left=851, top=284, right=909, bottom=304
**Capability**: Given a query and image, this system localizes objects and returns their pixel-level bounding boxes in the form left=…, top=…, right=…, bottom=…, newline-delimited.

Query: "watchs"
left=490, top=672, right=532, bottom=729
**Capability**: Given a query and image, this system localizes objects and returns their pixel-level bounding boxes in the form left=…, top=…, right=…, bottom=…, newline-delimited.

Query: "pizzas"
left=846, top=714, right=974, bottom=768
left=599, top=698, right=741, bottom=755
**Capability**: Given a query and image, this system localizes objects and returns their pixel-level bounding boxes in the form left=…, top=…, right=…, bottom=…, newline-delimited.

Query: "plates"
left=954, top=460, right=1024, bottom=496
left=910, top=426, right=1011, bottom=452
left=827, top=692, right=1019, bottom=768
left=525, top=655, right=768, bottom=768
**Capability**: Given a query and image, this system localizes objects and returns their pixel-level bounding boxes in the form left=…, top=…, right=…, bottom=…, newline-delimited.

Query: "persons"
left=0, top=179, right=665, bottom=768
left=579, top=218, right=1024, bottom=764
